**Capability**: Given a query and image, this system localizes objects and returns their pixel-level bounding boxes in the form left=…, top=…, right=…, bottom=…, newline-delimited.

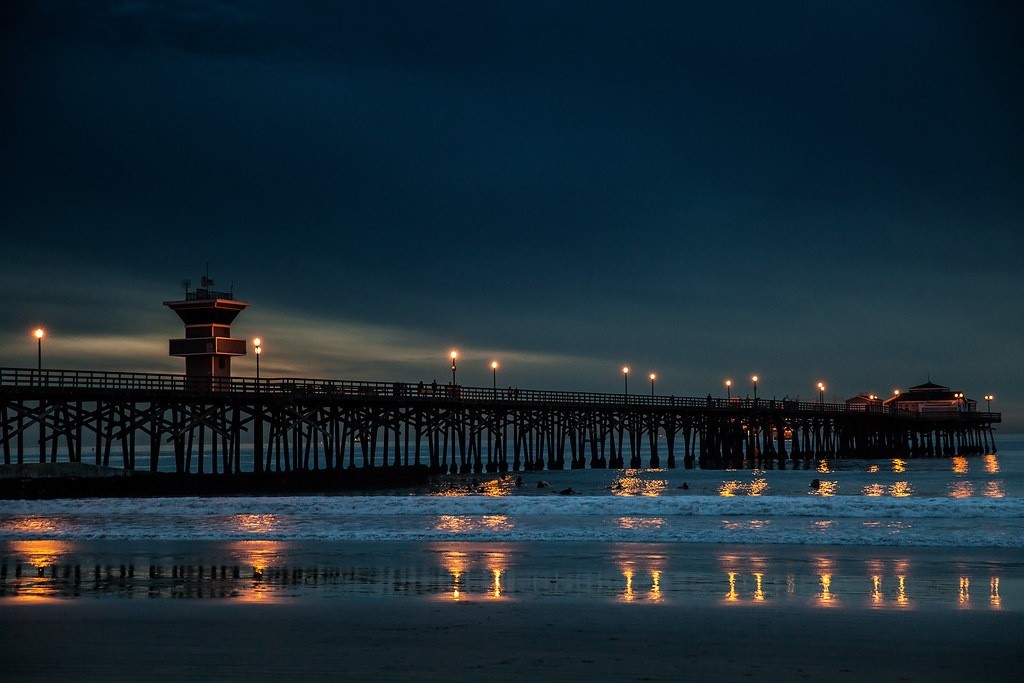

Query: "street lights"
left=955, top=393, right=963, bottom=405
left=894, top=390, right=899, bottom=414
left=492, top=361, right=497, bottom=400
left=254, top=338, right=261, bottom=392
left=985, top=395, right=993, bottom=412
left=451, top=351, right=457, bottom=398
left=35, top=329, right=43, bottom=386
left=752, top=376, right=758, bottom=401
left=821, top=387, right=824, bottom=411
left=726, top=381, right=730, bottom=405
left=623, top=367, right=629, bottom=404
left=650, top=374, right=655, bottom=405
left=818, top=383, right=822, bottom=412
